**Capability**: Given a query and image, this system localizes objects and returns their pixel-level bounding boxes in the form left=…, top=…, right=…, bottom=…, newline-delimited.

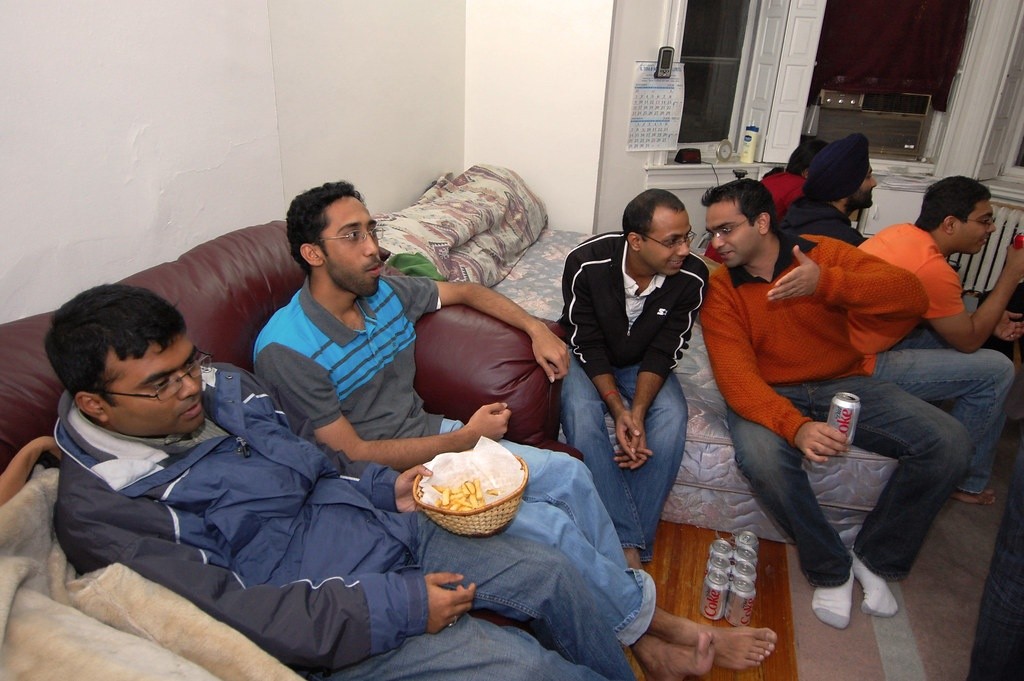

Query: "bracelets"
left=602, top=388, right=620, bottom=402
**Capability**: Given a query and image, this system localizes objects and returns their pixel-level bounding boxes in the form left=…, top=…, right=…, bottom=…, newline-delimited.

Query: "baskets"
left=413, top=454, right=529, bottom=538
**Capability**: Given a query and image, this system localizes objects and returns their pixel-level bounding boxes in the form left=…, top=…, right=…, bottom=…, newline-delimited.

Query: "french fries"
left=430, top=478, right=503, bottom=511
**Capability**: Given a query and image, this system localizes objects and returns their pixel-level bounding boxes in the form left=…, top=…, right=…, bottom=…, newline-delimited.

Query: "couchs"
left=0, top=219, right=584, bottom=681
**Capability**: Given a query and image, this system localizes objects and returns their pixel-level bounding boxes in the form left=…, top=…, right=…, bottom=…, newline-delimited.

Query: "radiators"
left=946, top=204, right=1024, bottom=292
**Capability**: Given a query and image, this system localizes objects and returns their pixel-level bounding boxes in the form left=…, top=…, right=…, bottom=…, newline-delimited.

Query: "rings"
left=1011, top=335, right=1013, bottom=337
left=449, top=615, right=457, bottom=628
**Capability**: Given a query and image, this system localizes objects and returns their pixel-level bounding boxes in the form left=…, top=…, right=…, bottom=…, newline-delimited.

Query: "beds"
left=491, top=227, right=899, bottom=551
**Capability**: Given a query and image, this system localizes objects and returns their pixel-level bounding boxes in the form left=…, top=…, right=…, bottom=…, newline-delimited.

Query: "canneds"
left=701, top=531, right=759, bottom=627
left=826, top=392, right=860, bottom=445
left=1013, top=232, right=1024, bottom=249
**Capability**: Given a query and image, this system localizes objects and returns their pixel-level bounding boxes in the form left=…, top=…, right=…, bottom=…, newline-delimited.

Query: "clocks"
left=715, top=139, right=733, bottom=162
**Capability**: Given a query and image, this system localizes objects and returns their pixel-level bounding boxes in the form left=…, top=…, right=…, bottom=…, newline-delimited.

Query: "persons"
left=760, top=131, right=877, bottom=247
left=560, top=189, right=710, bottom=570
left=849, top=176, right=1024, bottom=505
left=45, top=285, right=634, bottom=681
left=699, top=178, right=973, bottom=628
left=252, top=183, right=777, bottom=681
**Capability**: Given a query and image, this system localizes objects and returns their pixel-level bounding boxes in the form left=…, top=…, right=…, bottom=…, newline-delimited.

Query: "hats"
left=801, top=132, right=869, bottom=202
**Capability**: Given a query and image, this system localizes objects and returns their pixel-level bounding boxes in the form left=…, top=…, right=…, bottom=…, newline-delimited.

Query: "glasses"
left=98, top=349, right=212, bottom=402
left=702, top=217, right=749, bottom=241
left=963, top=217, right=996, bottom=225
left=641, top=230, right=697, bottom=250
left=318, top=226, right=386, bottom=243
